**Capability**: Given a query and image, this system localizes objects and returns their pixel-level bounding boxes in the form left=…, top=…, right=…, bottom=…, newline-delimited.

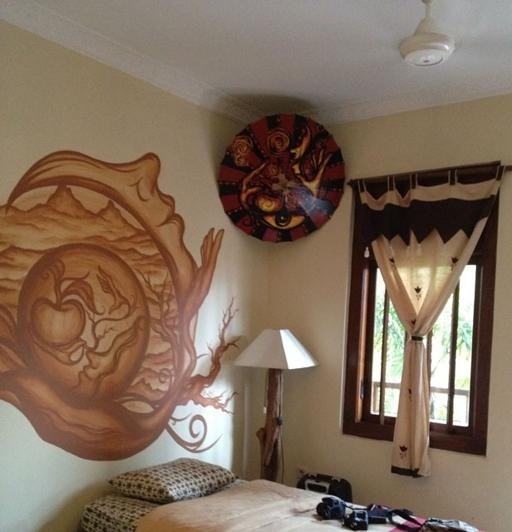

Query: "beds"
left=78, top=478, right=481, bottom=532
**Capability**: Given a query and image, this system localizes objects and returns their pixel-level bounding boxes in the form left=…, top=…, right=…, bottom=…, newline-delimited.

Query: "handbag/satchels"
left=419, top=518, right=478, bottom=532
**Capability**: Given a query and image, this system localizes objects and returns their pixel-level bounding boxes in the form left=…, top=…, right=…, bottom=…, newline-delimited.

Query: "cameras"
left=316, top=497, right=346, bottom=522
left=343, top=509, right=369, bottom=530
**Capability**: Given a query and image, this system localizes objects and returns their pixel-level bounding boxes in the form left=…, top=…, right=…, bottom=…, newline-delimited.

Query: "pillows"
left=105, top=457, right=240, bottom=504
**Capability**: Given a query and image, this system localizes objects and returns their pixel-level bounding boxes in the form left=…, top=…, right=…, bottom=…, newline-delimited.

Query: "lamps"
left=232, top=327, right=321, bottom=481
